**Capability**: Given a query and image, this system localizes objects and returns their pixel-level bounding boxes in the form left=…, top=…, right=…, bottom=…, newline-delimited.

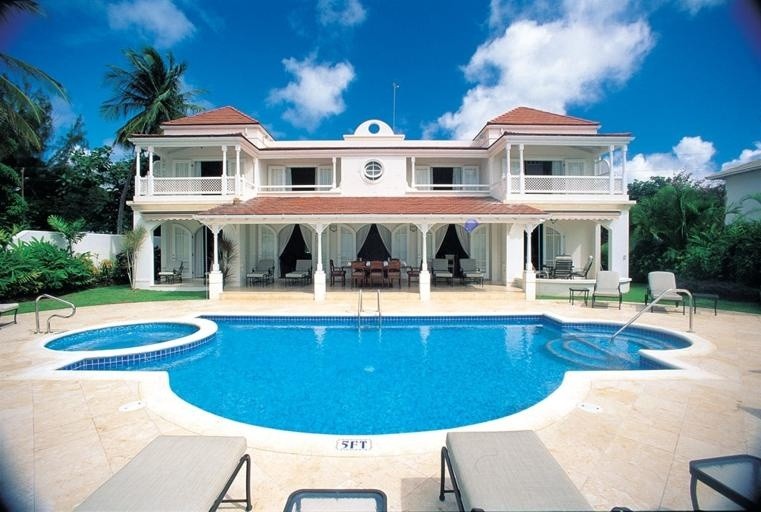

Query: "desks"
left=568, top=286, right=591, bottom=306
left=688, top=451, right=760, bottom=510
left=280, top=487, right=388, bottom=512
left=692, top=293, right=720, bottom=316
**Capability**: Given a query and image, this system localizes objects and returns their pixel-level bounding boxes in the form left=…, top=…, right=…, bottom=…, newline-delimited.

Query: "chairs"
left=0, top=302, right=18, bottom=327
left=523, top=252, right=594, bottom=281
left=328, top=256, right=423, bottom=288
left=592, top=268, right=632, bottom=309
left=437, top=428, right=630, bottom=511
left=430, top=253, right=486, bottom=288
left=73, top=432, right=254, bottom=511
left=246, top=258, right=275, bottom=286
left=645, top=270, right=686, bottom=315
left=159, top=260, right=185, bottom=285
left=285, top=258, right=313, bottom=287
